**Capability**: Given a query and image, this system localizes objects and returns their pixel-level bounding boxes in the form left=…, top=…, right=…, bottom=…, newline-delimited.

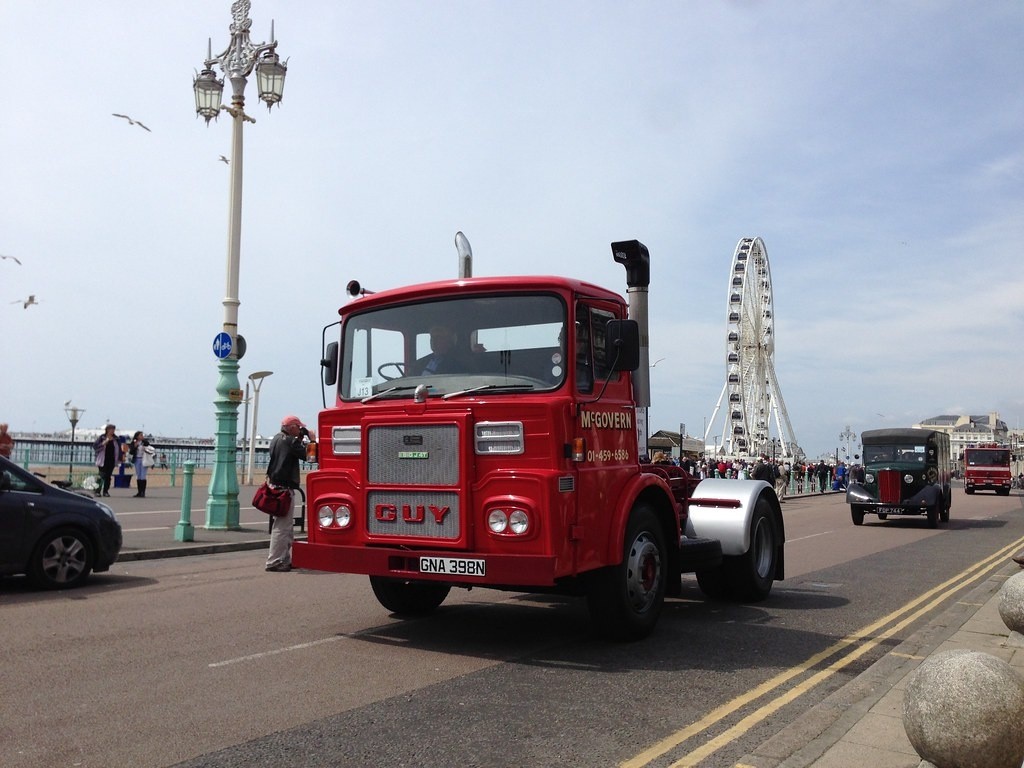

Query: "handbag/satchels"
left=252, top=482, right=292, bottom=518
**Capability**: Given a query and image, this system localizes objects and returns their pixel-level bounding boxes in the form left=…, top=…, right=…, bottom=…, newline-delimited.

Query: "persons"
left=160, top=453, right=168, bottom=471
left=833, top=461, right=845, bottom=490
left=93, top=425, right=122, bottom=496
left=264, top=415, right=318, bottom=572
left=814, top=460, right=831, bottom=493
left=680, top=457, right=690, bottom=472
left=671, top=456, right=772, bottom=479
left=752, top=456, right=774, bottom=488
left=877, top=449, right=892, bottom=459
left=557, top=322, right=587, bottom=358
left=775, top=460, right=787, bottom=502
left=652, top=452, right=670, bottom=465
left=785, top=459, right=862, bottom=487
left=1019, top=473, right=1023, bottom=478
left=130, top=431, right=153, bottom=497
left=408, top=321, right=480, bottom=376
left=0, top=423, right=14, bottom=459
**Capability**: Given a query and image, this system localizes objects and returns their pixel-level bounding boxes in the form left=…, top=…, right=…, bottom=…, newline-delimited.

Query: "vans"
left=846, top=428, right=952, bottom=528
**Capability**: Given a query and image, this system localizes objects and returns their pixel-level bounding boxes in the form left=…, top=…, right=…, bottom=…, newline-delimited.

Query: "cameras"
left=299, top=428, right=308, bottom=434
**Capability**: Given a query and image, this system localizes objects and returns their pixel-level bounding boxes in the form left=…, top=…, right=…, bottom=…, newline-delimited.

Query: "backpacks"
left=774, top=465, right=782, bottom=479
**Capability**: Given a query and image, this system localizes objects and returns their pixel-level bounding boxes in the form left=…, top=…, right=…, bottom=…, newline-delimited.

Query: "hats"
left=282, top=416, right=306, bottom=427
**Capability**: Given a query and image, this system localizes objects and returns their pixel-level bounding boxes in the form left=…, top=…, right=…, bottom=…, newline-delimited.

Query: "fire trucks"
left=963, top=443, right=1012, bottom=496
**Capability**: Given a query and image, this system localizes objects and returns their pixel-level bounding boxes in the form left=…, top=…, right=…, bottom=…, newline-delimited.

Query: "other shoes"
left=288, top=563, right=300, bottom=569
left=265, top=565, right=291, bottom=572
left=94, top=492, right=101, bottom=497
left=103, top=493, right=110, bottom=497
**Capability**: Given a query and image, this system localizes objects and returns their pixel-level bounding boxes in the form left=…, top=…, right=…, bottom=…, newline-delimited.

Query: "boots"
left=133, top=479, right=146, bottom=497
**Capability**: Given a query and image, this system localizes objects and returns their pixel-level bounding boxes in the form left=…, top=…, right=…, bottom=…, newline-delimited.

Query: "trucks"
left=289, top=231, right=785, bottom=642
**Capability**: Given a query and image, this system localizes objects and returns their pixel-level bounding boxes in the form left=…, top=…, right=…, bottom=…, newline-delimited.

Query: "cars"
left=0, top=455, right=122, bottom=590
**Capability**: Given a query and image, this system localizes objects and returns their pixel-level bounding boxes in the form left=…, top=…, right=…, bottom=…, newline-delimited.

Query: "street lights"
left=840, top=425, right=856, bottom=463
left=712, top=436, right=720, bottom=461
left=63, top=400, right=85, bottom=485
left=770, top=437, right=777, bottom=464
left=191, top=0, right=291, bottom=531
left=245, top=372, right=272, bottom=490
